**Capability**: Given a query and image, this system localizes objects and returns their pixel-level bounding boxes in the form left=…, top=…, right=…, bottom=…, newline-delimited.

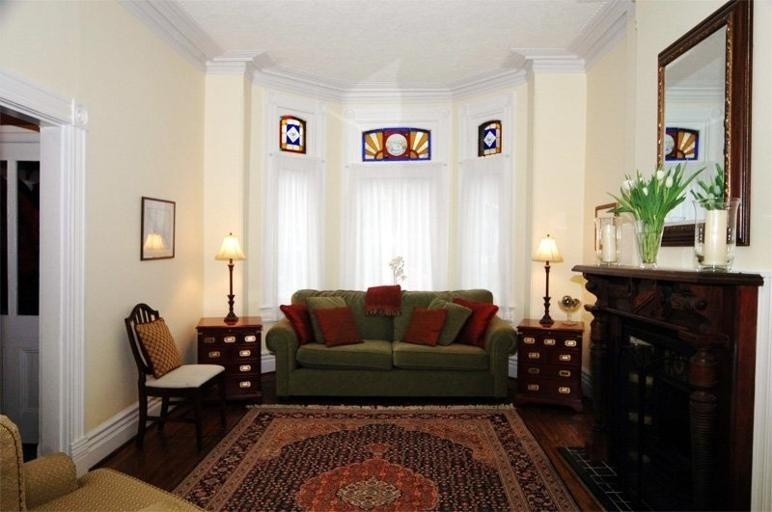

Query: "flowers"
left=604, top=157, right=709, bottom=267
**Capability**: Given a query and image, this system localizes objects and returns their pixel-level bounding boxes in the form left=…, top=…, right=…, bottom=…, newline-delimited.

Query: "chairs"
left=124, top=302, right=228, bottom=452
left=1, top=411, right=210, bottom=511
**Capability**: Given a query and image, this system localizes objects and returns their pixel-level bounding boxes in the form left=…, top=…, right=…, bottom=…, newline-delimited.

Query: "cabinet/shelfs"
left=194, top=314, right=264, bottom=406
left=515, top=318, right=586, bottom=413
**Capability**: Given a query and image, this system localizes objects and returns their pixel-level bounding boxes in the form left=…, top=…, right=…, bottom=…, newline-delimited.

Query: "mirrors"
left=655, top=1, right=753, bottom=249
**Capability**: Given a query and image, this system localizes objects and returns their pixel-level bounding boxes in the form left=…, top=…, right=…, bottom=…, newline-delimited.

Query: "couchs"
left=266, top=287, right=518, bottom=398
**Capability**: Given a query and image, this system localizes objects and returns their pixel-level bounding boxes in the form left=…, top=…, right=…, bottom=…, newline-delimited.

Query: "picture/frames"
left=593, top=201, right=620, bottom=253
left=140, top=196, right=177, bottom=261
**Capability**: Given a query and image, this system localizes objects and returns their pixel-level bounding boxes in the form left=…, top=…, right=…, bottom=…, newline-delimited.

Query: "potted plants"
left=690, top=162, right=741, bottom=276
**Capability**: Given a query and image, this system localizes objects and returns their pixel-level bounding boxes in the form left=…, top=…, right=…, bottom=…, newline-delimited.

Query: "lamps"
left=143, top=233, right=167, bottom=252
left=214, top=232, right=246, bottom=321
left=530, top=233, right=564, bottom=326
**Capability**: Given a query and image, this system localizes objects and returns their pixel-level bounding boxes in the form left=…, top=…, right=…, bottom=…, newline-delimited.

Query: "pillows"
left=279, top=303, right=315, bottom=345
left=425, top=295, right=472, bottom=347
left=136, top=316, right=183, bottom=380
left=451, top=296, right=499, bottom=348
left=305, top=295, right=348, bottom=344
left=313, top=302, right=362, bottom=348
left=401, top=306, right=449, bottom=347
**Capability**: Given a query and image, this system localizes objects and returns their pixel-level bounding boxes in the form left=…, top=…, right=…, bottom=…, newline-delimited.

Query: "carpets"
left=171, top=402, right=583, bottom=512
left=554, top=442, right=650, bottom=510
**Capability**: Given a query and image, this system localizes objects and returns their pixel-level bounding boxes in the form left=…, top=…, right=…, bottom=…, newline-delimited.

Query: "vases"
left=597, top=211, right=619, bottom=266
left=633, top=219, right=665, bottom=270
